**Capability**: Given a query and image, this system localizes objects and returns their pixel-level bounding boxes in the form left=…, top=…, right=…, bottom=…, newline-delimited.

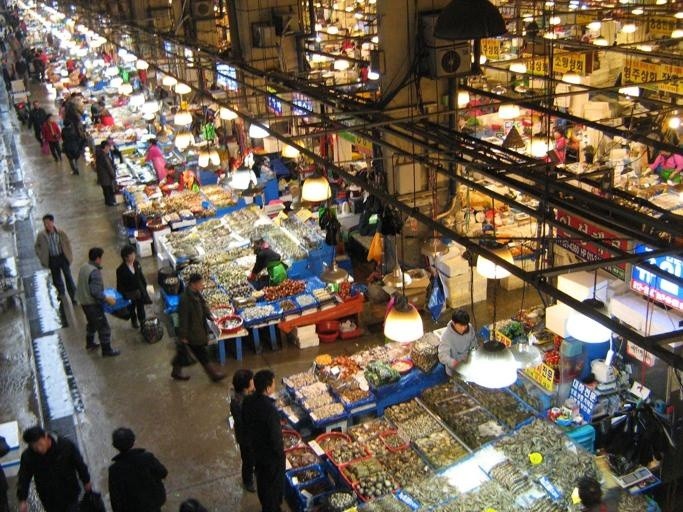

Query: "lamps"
left=249, top=119, right=271, bottom=140
left=173, top=125, right=195, bottom=151
left=196, top=147, right=221, bottom=171
left=299, top=161, right=331, bottom=203
left=477, top=238, right=514, bottom=279
left=172, top=108, right=195, bottom=126
left=161, top=74, right=178, bottom=87
left=464, top=342, right=519, bottom=392
left=381, top=292, right=426, bottom=348
left=564, top=297, right=612, bottom=347
left=227, top=168, right=257, bottom=191
left=218, top=100, right=239, bottom=122
left=434, top=1, right=506, bottom=40
left=173, top=80, right=191, bottom=95
left=142, top=99, right=160, bottom=115
left=279, top=133, right=307, bottom=159
left=127, top=88, right=145, bottom=109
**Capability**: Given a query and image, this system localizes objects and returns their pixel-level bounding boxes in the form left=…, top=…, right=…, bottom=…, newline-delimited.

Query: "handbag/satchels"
left=124, top=290, right=141, bottom=302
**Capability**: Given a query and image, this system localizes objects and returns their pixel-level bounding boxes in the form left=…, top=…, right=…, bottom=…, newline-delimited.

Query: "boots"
left=172, top=365, right=190, bottom=381
left=101, top=342, right=120, bottom=356
left=86, top=336, right=100, bottom=348
left=204, top=362, right=228, bottom=382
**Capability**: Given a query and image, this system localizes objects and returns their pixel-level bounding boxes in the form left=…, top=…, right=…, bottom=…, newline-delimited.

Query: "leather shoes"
left=246, top=484, right=256, bottom=492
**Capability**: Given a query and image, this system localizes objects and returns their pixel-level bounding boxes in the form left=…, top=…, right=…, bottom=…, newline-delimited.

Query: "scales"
left=608, top=463, right=653, bottom=488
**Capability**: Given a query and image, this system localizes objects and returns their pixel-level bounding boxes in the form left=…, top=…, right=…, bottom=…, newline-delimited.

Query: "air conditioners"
left=191, top=1, right=214, bottom=16
left=276, top=12, right=300, bottom=36
left=423, top=8, right=471, bottom=78
left=255, top=24, right=276, bottom=47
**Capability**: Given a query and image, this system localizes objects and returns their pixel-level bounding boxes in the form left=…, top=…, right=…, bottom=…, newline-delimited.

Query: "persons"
left=95, top=136, right=120, bottom=207
left=246, top=236, right=287, bottom=283
left=170, top=273, right=227, bottom=384
left=116, top=246, right=151, bottom=328
left=546, top=129, right=566, bottom=163
left=16, top=425, right=91, bottom=511
left=0, top=435, right=11, bottom=512
left=1, top=1, right=48, bottom=90
left=27, top=93, right=113, bottom=176
left=179, top=498, right=209, bottom=512
left=436, top=309, right=480, bottom=380
left=260, top=156, right=274, bottom=182
left=140, top=138, right=169, bottom=182
left=243, top=370, right=288, bottom=512
left=572, top=474, right=609, bottom=512
left=640, top=149, right=683, bottom=183
left=154, top=87, right=167, bottom=99
left=76, top=247, right=124, bottom=357
left=228, top=372, right=255, bottom=494
left=35, top=213, right=80, bottom=306
left=108, top=427, right=168, bottom=512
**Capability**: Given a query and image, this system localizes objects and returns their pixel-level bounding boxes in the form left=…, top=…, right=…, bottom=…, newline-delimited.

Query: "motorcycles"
left=15, top=98, right=34, bottom=122
